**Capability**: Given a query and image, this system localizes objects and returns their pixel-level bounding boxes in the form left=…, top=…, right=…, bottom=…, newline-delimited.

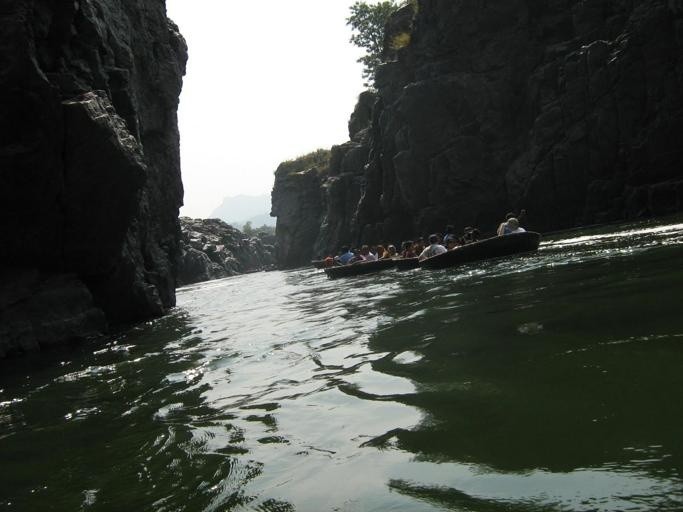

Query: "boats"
left=310, top=230, right=550, bottom=280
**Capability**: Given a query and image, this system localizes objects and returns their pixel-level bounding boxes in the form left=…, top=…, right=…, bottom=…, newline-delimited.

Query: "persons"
left=312, top=205, right=532, bottom=276
